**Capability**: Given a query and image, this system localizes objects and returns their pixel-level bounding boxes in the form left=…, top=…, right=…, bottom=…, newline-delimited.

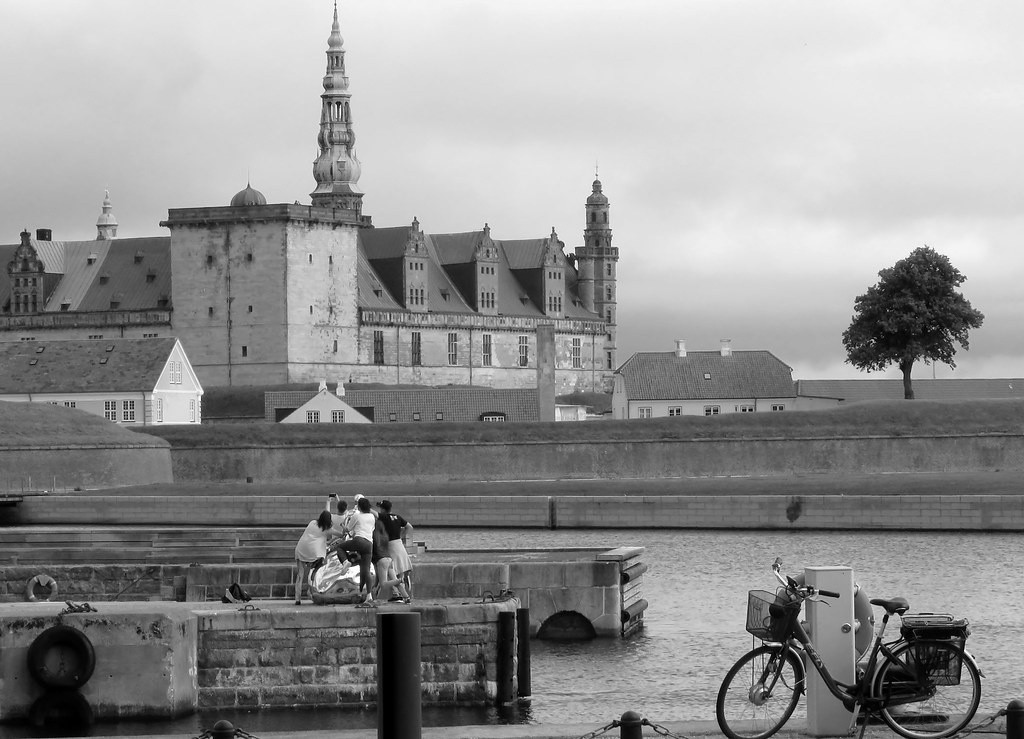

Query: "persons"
left=294, top=492, right=414, bottom=606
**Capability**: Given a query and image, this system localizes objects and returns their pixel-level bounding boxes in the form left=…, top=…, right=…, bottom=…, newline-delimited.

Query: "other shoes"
left=296, top=600, right=301, bottom=605
left=403, top=596, right=410, bottom=604
left=388, top=596, right=403, bottom=602
left=404, top=575, right=411, bottom=591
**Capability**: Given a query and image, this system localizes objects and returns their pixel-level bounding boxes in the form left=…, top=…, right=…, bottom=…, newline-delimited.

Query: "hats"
left=376, top=500, right=392, bottom=510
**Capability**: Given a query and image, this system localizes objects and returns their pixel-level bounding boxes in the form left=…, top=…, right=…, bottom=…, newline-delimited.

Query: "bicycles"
left=716, top=557, right=986, bottom=739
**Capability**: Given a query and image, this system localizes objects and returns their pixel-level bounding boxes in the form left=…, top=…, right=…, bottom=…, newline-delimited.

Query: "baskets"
left=901, top=613, right=954, bottom=669
left=746, top=589, right=799, bottom=641
left=913, top=629, right=967, bottom=686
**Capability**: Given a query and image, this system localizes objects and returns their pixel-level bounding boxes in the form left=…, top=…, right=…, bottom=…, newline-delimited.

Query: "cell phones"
left=329, top=493, right=336, bottom=497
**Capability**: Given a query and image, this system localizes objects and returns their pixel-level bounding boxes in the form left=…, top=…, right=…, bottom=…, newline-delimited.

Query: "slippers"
left=355, top=602, right=378, bottom=608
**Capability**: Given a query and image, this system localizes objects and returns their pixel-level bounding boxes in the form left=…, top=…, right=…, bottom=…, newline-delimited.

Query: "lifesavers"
left=26, top=625, right=97, bottom=689
left=770, top=573, right=876, bottom=673
left=26, top=688, right=95, bottom=738
left=26, top=574, right=60, bottom=602
